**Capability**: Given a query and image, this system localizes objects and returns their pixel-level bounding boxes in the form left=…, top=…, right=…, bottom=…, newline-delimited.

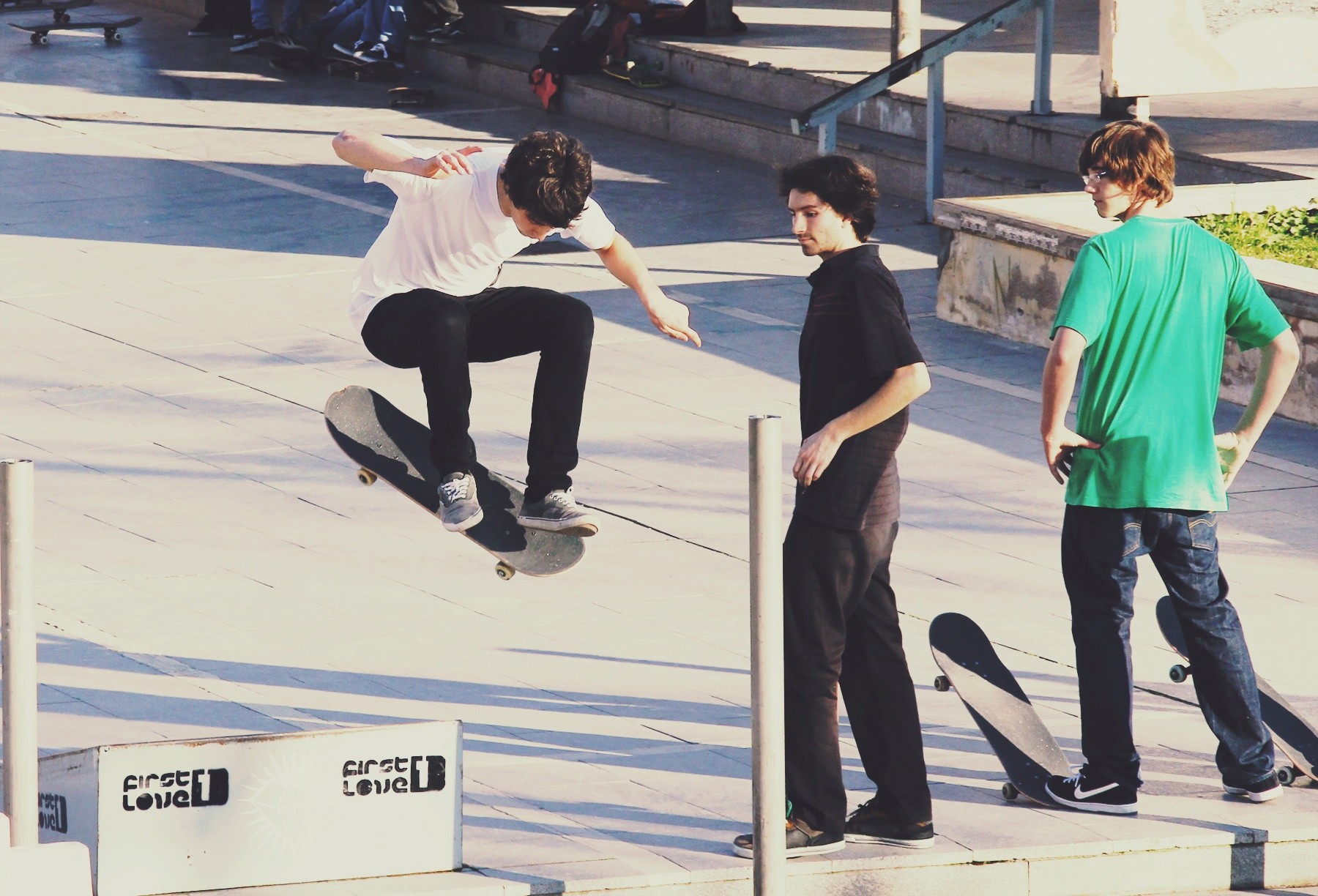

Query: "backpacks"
left=527, top=0, right=635, bottom=88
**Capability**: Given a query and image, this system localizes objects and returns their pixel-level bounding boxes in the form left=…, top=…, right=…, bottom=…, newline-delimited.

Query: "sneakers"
left=1219, top=769, right=1284, bottom=802
left=841, top=798, right=940, bottom=850
left=1044, top=764, right=1141, bottom=817
left=437, top=468, right=484, bottom=531
left=729, top=812, right=849, bottom=860
left=514, top=486, right=599, bottom=536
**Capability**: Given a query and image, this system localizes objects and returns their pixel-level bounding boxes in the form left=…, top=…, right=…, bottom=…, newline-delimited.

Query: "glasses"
left=1081, top=170, right=1109, bottom=187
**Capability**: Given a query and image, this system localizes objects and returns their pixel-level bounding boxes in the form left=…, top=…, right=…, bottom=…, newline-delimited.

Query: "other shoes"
left=354, top=43, right=393, bottom=64
left=229, top=29, right=267, bottom=55
left=187, top=21, right=212, bottom=36
left=332, top=38, right=373, bottom=59
left=427, top=24, right=445, bottom=36
left=431, top=22, right=467, bottom=46
left=269, top=52, right=323, bottom=73
left=232, top=32, right=269, bottom=44
left=274, top=35, right=310, bottom=53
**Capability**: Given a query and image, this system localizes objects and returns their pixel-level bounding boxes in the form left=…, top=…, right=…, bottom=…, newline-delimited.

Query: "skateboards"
left=1156, top=593, right=1318, bottom=786
left=388, top=86, right=433, bottom=107
left=6, top=16, right=144, bottom=46
left=603, top=54, right=668, bottom=88
left=927, top=611, right=1070, bottom=810
left=325, top=383, right=587, bottom=578
left=321, top=35, right=395, bottom=79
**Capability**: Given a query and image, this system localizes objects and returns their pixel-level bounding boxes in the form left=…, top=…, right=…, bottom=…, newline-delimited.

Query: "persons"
left=186, top=0, right=745, bottom=111
left=733, top=153, right=932, bottom=861
left=332, top=124, right=704, bottom=535
left=1039, top=119, right=1300, bottom=817
